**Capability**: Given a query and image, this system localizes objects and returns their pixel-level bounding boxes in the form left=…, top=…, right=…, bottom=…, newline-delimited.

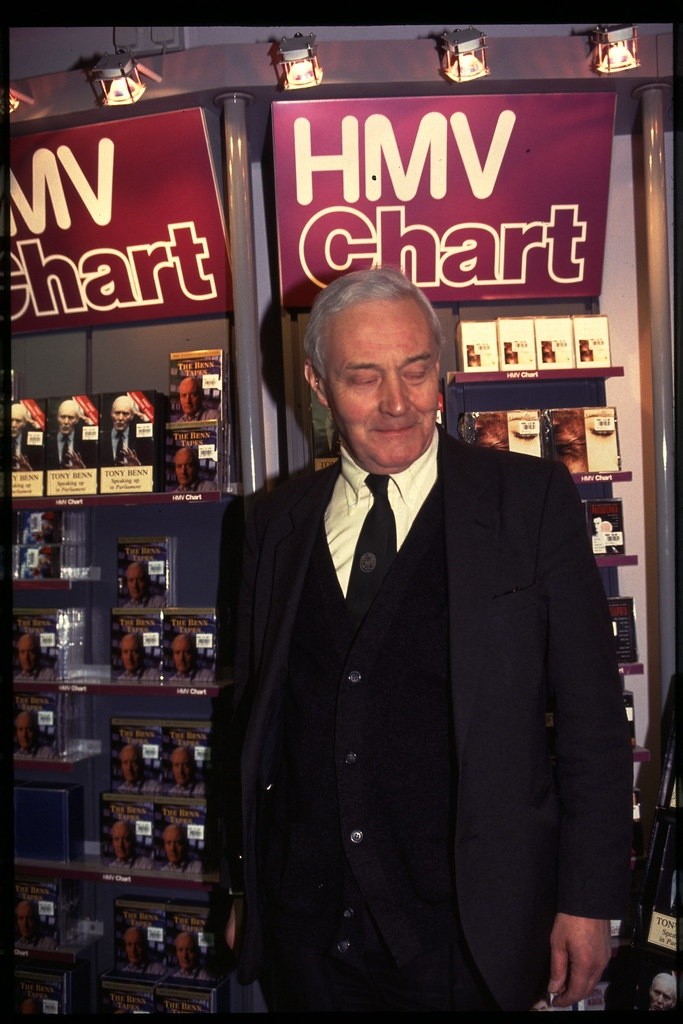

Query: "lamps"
left=92, top=49, right=163, bottom=107
left=275, top=33, right=323, bottom=92
left=440, top=27, right=491, bottom=84
left=593, top=23, right=643, bottom=76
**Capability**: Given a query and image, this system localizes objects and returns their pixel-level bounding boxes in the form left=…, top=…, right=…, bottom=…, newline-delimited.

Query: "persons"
left=224, top=269, right=634, bottom=1011
left=647, top=972, right=677, bottom=1010
left=12, top=377, right=219, bottom=1014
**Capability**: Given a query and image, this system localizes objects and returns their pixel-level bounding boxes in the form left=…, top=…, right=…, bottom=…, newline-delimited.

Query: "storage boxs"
left=460, top=316, right=637, bottom=847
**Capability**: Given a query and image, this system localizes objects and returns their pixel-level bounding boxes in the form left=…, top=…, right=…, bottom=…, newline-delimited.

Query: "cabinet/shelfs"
left=290, top=303, right=652, bottom=1012
left=10, top=305, right=248, bottom=1014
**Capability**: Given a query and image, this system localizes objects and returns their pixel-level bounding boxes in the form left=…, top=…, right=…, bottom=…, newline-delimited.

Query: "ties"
left=115, top=432, right=126, bottom=466
left=12, top=439, right=19, bottom=467
left=60, top=435, right=71, bottom=467
left=345, top=473, right=397, bottom=627
